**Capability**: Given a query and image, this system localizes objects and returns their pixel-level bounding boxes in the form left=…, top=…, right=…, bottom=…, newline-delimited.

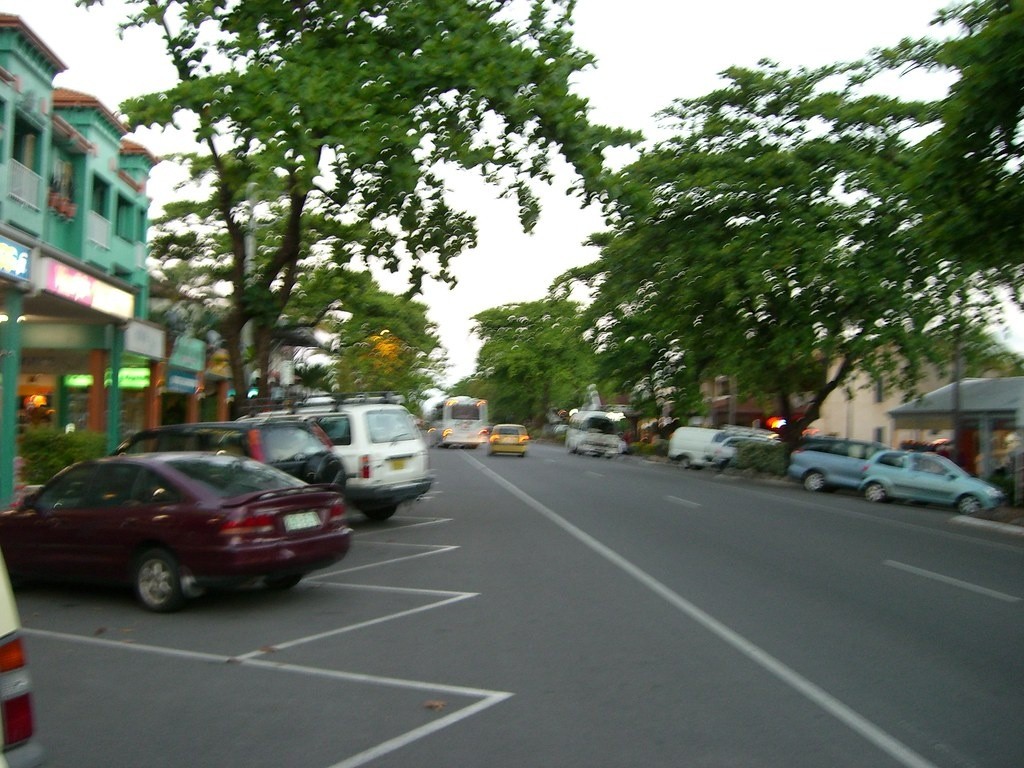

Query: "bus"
left=567, top=408, right=618, bottom=459
left=442, top=394, right=488, bottom=449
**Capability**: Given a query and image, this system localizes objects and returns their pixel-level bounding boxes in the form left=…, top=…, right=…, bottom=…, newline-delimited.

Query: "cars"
left=490, top=424, right=530, bottom=455
left=0, top=450, right=355, bottom=612
left=786, top=437, right=897, bottom=494
left=858, top=451, right=1007, bottom=517
left=705, top=433, right=782, bottom=469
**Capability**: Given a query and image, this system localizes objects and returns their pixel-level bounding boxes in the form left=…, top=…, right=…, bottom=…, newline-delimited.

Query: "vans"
left=669, top=427, right=718, bottom=469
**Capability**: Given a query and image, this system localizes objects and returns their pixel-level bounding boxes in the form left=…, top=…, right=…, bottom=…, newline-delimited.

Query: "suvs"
left=230, top=387, right=436, bottom=522
left=105, top=417, right=348, bottom=494
